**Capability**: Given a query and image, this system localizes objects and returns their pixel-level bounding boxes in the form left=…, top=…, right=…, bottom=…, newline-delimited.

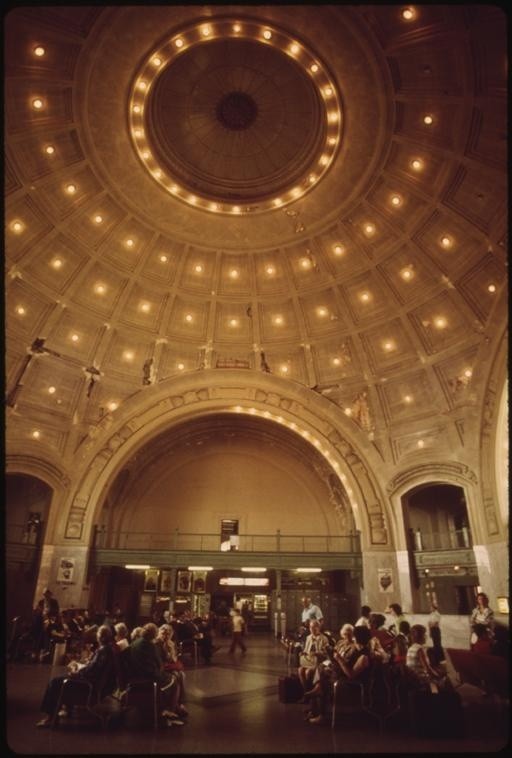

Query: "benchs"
left=322, top=662, right=444, bottom=731
left=51, top=644, right=160, bottom=731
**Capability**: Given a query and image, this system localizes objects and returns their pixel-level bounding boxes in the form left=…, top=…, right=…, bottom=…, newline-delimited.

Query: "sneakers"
left=36, top=718, right=61, bottom=728
left=161, top=704, right=189, bottom=727
left=303, top=688, right=329, bottom=725
left=58, top=703, right=77, bottom=716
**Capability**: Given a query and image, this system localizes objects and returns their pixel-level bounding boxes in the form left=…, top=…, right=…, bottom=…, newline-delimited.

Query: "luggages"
left=279, top=642, right=304, bottom=704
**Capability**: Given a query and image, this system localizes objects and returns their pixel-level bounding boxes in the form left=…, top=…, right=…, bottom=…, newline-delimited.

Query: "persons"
left=16, top=585, right=221, bottom=730
left=241, top=599, right=248, bottom=614
left=296, top=595, right=478, bottom=725
left=409, top=527, right=417, bottom=550
left=248, top=600, right=253, bottom=612
left=469, top=591, right=495, bottom=650
left=472, top=621, right=493, bottom=652
left=228, top=608, right=248, bottom=654
left=415, top=527, right=423, bottom=551
left=29, top=512, right=40, bottom=545
left=492, top=623, right=511, bottom=659
left=21, top=512, right=32, bottom=543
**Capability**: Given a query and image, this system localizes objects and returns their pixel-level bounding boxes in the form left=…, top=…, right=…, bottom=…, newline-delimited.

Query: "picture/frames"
left=143, top=569, right=208, bottom=595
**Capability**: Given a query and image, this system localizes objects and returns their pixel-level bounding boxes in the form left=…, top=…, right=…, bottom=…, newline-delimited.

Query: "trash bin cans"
left=274, top=610, right=286, bottom=637
left=220, top=518, right=239, bottom=551
